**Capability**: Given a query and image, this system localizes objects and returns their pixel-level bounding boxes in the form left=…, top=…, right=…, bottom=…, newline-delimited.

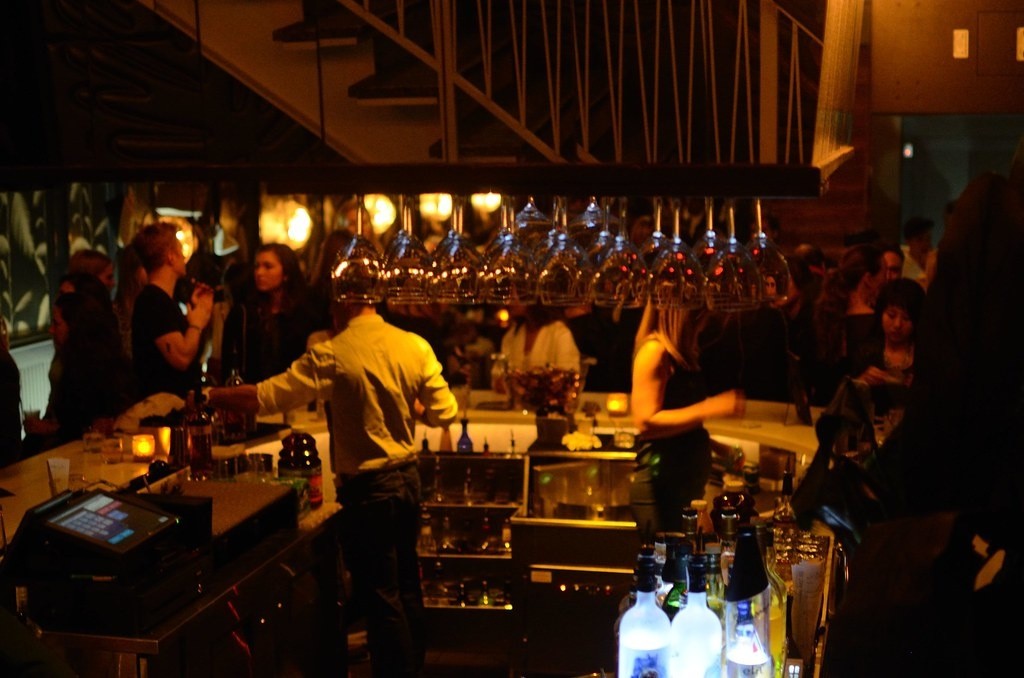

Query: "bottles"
left=616, top=466, right=797, bottom=678
left=171, top=367, right=323, bottom=509
left=417, top=411, right=522, bottom=607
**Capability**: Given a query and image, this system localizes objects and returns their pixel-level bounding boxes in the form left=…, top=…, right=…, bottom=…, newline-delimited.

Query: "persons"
left=185, top=256, right=460, bottom=678
left=631, top=271, right=746, bottom=579
left=491, top=196, right=806, bottom=400
left=0, top=232, right=388, bottom=467
left=753, top=218, right=1024, bottom=678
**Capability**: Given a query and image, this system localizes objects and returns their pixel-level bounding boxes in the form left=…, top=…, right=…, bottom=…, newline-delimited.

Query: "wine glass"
left=331, top=195, right=794, bottom=311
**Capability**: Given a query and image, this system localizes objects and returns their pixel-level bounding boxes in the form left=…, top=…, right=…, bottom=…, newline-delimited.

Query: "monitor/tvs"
left=40, top=488, right=182, bottom=555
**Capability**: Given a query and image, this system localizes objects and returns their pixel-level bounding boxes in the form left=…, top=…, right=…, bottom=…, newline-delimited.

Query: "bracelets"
left=188, top=324, right=202, bottom=332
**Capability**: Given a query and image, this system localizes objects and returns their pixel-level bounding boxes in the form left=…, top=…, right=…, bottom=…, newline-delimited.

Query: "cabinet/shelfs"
left=869, top=112, right=1024, bottom=247
left=420, top=450, right=638, bottom=615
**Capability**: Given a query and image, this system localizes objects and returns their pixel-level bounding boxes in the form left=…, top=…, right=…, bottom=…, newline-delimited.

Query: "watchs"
left=202, top=386, right=215, bottom=406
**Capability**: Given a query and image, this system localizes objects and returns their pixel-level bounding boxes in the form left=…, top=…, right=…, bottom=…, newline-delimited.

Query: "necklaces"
left=881, top=349, right=909, bottom=374
